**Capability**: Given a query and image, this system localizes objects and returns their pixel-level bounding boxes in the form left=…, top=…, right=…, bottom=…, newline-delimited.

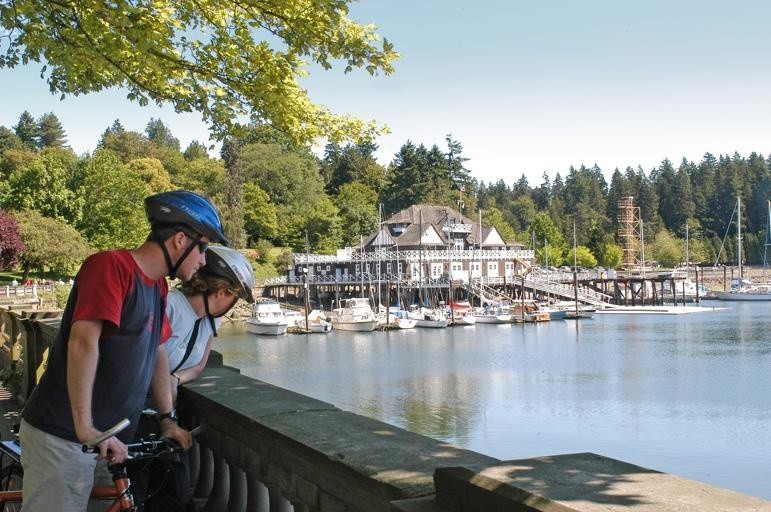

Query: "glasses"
left=185, top=233, right=208, bottom=254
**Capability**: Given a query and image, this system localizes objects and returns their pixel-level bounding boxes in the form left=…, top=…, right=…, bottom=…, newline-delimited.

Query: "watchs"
left=170, top=372, right=181, bottom=386
left=159, top=409, right=180, bottom=422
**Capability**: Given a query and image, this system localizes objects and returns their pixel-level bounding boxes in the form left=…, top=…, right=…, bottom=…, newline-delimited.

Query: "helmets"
left=144, top=191, right=228, bottom=247
left=207, top=246, right=255, bottom=304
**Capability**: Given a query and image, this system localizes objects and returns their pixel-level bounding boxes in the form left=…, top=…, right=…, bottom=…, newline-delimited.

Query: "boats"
left=284, top=203, right=597, bottom=335
left=245, top=298, right=288, bottom=336
left=656, top=197, right=771, bottom=300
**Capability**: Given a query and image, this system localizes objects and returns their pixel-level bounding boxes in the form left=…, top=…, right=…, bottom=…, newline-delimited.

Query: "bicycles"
left=0, top=404, right=207, bottom=512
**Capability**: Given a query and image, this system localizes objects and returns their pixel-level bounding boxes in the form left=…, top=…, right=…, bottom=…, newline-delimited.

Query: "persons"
left=125, top=247, right=255, bottom=512
left=19, top=190, right=230, bottom=512
left=11, top=276, right=74, bottom=286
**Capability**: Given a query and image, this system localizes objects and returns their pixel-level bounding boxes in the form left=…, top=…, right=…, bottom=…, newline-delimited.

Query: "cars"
left=535, top=265, right=605, bottom=273
